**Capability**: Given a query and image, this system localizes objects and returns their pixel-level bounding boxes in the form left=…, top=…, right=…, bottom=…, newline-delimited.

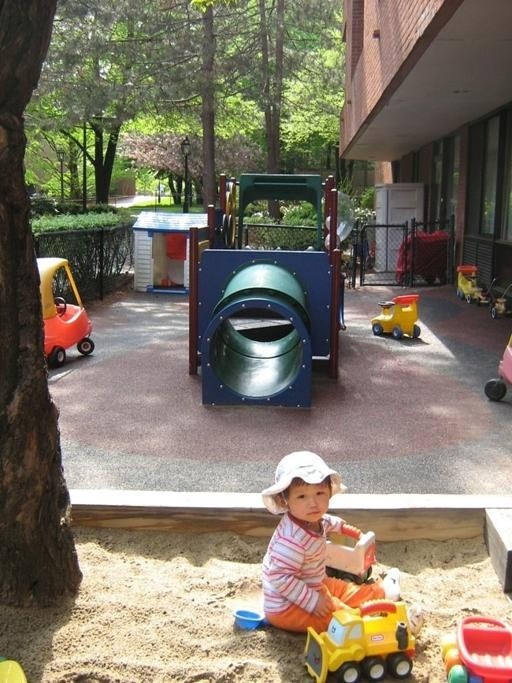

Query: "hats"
left=262, top=452, right=349, bottom=516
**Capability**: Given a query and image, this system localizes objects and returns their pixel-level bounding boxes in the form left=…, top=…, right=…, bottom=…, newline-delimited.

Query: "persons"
left=261, top=450, right=422, bottom=638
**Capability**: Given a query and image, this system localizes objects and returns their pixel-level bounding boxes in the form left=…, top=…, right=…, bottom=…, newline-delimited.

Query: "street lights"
left=55, top=147, right=66, bottom=202
left=180, top=133, right=193, bottom=212
